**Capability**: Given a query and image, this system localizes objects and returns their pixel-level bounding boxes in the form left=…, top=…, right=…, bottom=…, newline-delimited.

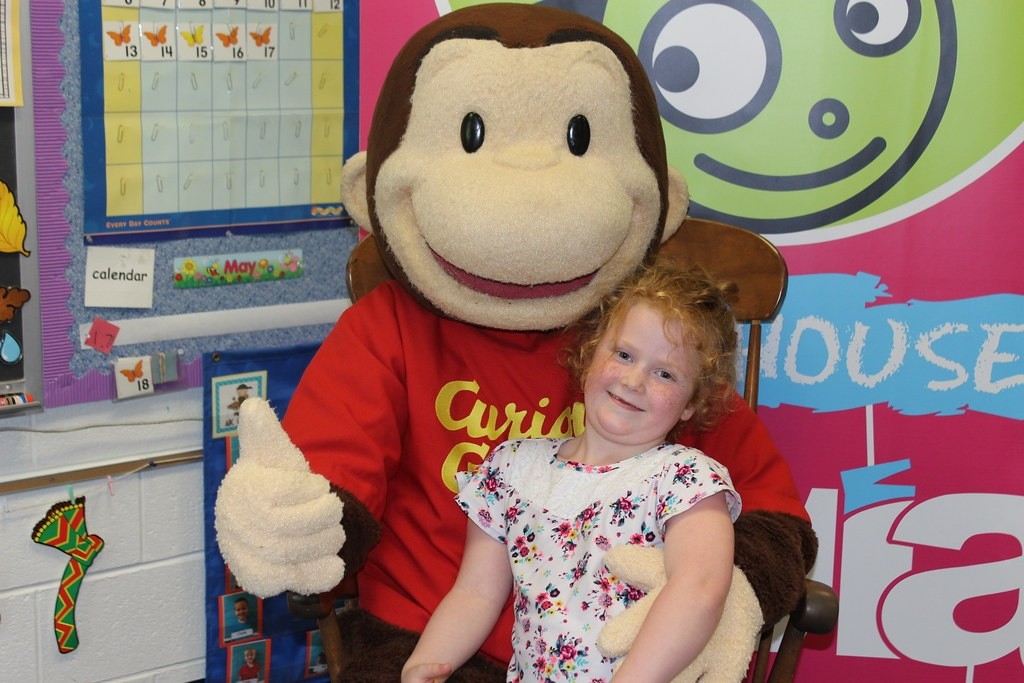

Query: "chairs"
left=287, top=218, right=838, bottom=683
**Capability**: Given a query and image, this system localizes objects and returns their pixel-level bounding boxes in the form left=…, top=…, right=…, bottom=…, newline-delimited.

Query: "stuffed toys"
left=213, top=2, right=817, bottom=683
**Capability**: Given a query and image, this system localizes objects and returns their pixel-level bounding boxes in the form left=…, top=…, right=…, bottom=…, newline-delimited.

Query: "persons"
left=400, top=260, right=743, bottom=683
